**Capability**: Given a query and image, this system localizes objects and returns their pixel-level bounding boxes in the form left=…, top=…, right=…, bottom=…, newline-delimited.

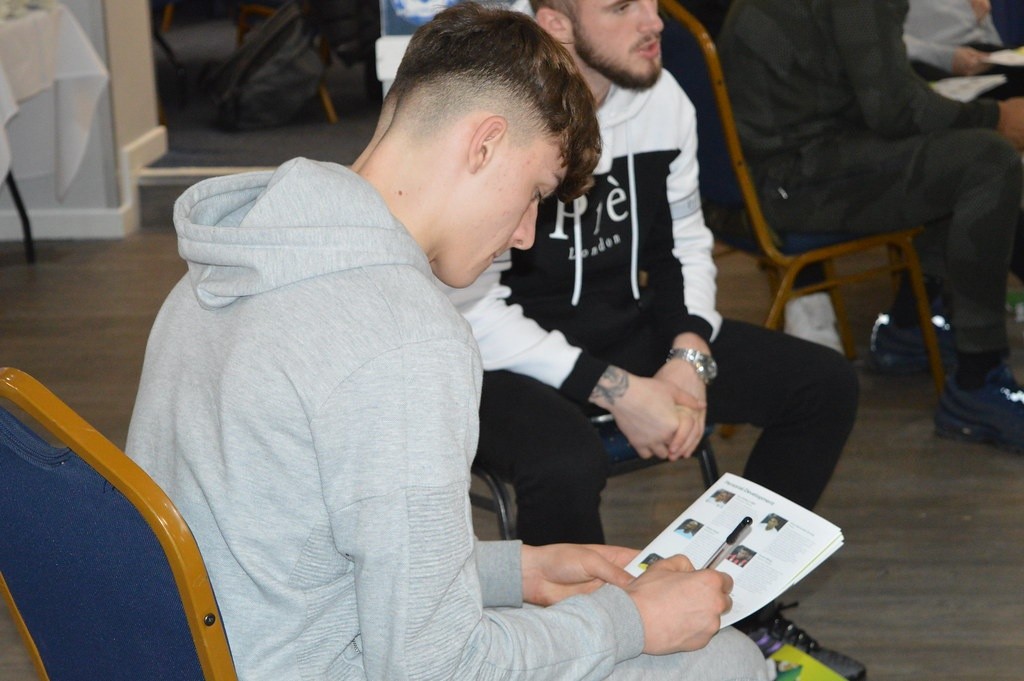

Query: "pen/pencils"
left=698, top=515, right=755, bottom=570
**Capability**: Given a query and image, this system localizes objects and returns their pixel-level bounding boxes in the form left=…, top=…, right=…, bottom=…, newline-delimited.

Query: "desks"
left=0, top=0, right=110, bottom=262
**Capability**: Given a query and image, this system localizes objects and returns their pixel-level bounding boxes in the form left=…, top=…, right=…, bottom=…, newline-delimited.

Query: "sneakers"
left=873, top=312, right=956, bottom=376
left=746, top=601, right=866, bottom=681
left=933, top=381, right=1024, bottom=445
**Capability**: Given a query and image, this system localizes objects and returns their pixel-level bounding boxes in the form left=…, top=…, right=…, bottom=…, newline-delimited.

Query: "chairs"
left=234, top=5, right=370, bottom=126
left=659, top=0, right=943, bottom=391
left=466, top=401, right=718, bottom=540
left=0, top=364, right=239, bottom=681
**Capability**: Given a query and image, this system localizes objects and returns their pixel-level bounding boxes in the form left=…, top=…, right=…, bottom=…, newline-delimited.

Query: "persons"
left=125, top=2, right=774, bottom=678
left=638, top=491, right=779, bottom=571
left=428, top=0, right=1024, bottom=552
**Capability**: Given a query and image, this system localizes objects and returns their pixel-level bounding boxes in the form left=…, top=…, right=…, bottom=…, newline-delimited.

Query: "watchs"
left=666, top=348, right=717, bottom=383
